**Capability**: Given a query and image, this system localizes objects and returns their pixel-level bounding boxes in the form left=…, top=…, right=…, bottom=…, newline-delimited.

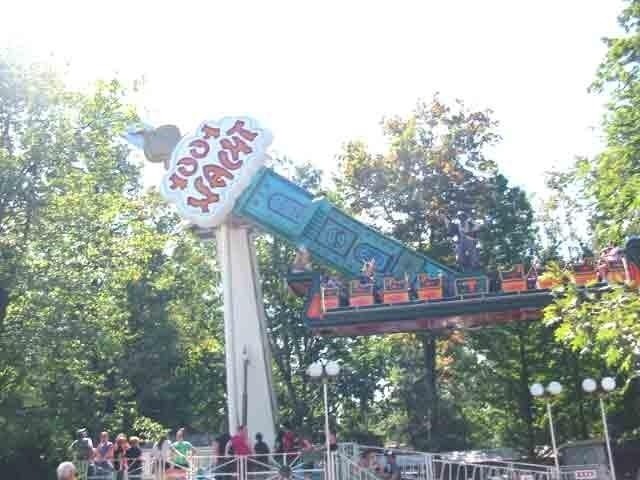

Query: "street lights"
left=302, top=356, right=343, bottom=480
left=527, top=380, right=566, bottom=480
left=580, top=375, right=618, bottom=480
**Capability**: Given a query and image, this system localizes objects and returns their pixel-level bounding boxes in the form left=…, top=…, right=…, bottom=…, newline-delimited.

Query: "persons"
left=56, top=427, right=198, bottom=480
left=594, top=239, right=620, bottom=285
left=320, top=257, right=383, bottom=303
left=211, top=423, right=340, bottom=480
left=352, top=448, right=401, bottom=480
